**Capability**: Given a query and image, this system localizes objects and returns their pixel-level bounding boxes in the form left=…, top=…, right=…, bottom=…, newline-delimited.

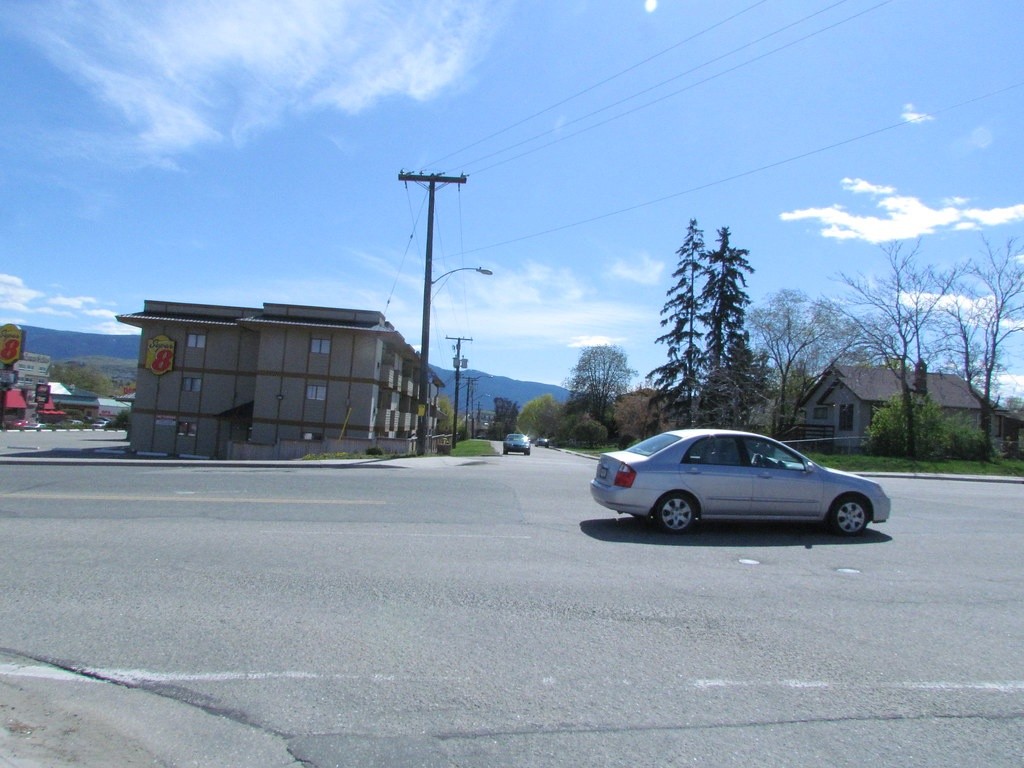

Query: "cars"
left=83, top=416, right=92, bottom=420
left=590, top=429, right=890, bottom=534
left=0, top=415, right=18, bottom=428
left=19, top=423, right=41, bottom=432
left=503, top=434, right=530, bottom=455
left=11, top=420, right=30, bottom=428
left=535, top=438, right=549, bottom=448
left=91, top=416, right=112, bottom=428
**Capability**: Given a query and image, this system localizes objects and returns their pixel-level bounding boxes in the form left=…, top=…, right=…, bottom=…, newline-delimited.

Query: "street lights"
left=415, top=267, right=493, bottom=456
left=451, top=375, right=493, bottom=448
left=465, top=394, right=490, bottom=439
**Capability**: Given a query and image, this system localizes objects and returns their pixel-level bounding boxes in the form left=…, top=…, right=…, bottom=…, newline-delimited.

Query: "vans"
left=69, top=420, right=84, bottom=425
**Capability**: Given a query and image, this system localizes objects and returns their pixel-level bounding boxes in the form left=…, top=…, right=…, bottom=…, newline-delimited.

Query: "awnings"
left=36, top=410, right=67, bottom=415
left=0, top=390, right=27, bottom=409
